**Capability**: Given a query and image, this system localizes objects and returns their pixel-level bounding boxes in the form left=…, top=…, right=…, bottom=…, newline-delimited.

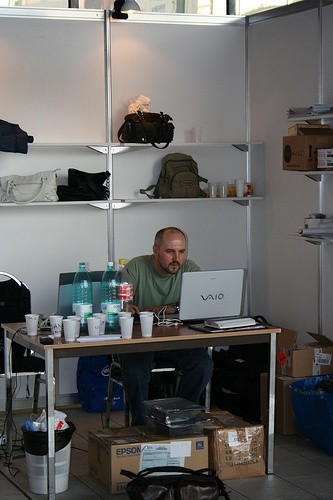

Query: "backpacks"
left=140, top=152, right=208, bottom=198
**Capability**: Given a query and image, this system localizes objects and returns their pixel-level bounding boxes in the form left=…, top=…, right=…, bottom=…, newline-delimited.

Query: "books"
left=202, top=315, right=258, bottom=329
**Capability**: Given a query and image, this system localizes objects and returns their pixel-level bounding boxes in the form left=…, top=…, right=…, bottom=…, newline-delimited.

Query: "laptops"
left=56, top=271, right=121, bottom=319
left=154, top=268, right=244, bottom=323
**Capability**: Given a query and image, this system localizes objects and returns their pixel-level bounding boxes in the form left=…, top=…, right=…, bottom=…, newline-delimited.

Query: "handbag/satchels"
left=67, top=166, right=111, bottom=199
left=117, top=110, right=175, bottom=149
left=119, top=465, right=231, bottom=500
left=0, top=168, right=61, bottom=204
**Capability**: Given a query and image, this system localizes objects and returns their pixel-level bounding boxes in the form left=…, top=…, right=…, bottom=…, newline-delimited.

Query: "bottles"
left=99, top=261, right=120, bottom=332
left=70, top=262, right=94, bottom=333
left=112, top=258, right=135, bottom=332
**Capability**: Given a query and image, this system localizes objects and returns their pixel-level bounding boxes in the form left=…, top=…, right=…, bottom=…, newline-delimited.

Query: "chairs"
left=0, top=272, right=56, bottom=465
left=105, top=345, right=213, bottom=429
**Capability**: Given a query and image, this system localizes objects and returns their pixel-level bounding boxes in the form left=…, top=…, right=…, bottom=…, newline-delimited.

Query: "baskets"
left=20, top=421, right=72, bottom=495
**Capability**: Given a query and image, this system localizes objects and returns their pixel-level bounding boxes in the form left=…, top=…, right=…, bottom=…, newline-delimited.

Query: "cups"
left=67, top=315, right=82, bottom=338
left=118, top=311, right=132, bottom=327
left=120, top=316, right=134, bottom=338
left=62, top=319, right=76, bottom=341
left=49, top=315, right=63, bottom=337
left=140, top=311, right=154, bottom=316
left=25, top=314, right=40, bottom=335
left=235, top=179, right=245, bottom=198
left=87, top=317, right=101, bottom=337
left=140, top=314, right=154, bottom=337
left=208, top=181, right=218, bottom=198
left=91, top=312, right=106, bottom=335
left=219, top=182, right=228, bottom=198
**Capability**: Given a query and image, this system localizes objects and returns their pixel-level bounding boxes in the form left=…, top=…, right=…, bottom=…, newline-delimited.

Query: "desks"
left=0, top=317, right=282, bottom=500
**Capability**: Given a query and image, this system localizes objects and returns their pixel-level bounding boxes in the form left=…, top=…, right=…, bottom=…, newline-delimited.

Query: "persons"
left=113, top=226, right=215, bottom=429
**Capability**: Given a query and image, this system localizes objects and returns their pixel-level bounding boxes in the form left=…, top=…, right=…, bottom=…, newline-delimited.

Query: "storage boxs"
left=276, top=327, right=333, bottom=378
left=141, top=397, right=212, bottom=437
left=259, top=372, right=306, bottom=436
left=203, top=406, right=266, bottom=482
left=86, top=424, right=209, bottom=495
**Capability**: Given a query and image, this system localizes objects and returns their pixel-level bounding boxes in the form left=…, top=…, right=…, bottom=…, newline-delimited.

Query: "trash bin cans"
left=21, top=421, right=75, bottom=495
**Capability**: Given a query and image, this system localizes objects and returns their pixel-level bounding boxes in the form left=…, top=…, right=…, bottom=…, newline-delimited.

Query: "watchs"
left=172, top=303, right=180, bottom=314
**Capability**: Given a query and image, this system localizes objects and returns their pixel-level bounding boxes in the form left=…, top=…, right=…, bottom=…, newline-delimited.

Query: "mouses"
left=131, top=313, right=140, bottom=324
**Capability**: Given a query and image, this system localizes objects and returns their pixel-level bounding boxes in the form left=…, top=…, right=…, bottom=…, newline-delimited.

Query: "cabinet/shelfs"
left=0, top=0, right=333, bottom=413
